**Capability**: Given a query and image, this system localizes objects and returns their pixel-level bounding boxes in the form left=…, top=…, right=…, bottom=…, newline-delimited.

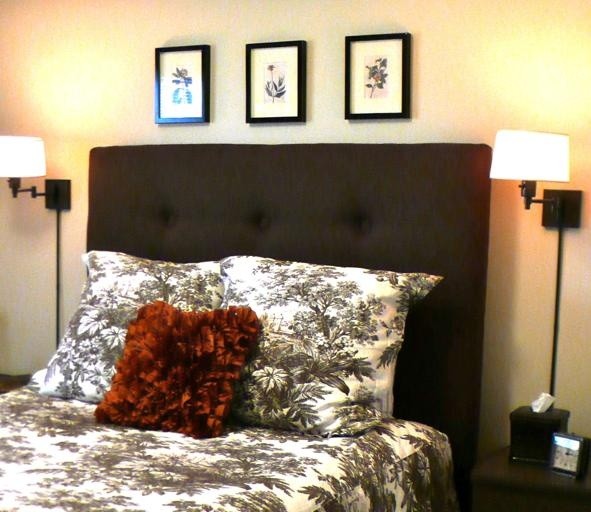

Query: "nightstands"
left=473, top=443, right=591, bottom=511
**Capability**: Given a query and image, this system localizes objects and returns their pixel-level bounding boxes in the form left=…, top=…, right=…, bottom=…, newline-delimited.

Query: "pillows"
left=218, top=254, right=443, bottom=436
left=36, top=250, right=223, bottom=401
left=95, top=303, right=260, bottom=439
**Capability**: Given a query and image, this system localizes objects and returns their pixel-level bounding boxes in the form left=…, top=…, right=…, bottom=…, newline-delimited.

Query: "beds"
left=0, top=142, right=491, bottom=512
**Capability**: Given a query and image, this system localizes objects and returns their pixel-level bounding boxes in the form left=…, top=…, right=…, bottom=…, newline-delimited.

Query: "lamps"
left=0, top=134, right=72, bottom=211
left=490, top=127, right=583, bottom=228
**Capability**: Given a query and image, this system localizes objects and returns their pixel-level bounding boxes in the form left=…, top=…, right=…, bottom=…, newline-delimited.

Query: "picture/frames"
left=245, top=40, right=308, bottom=124
left=154, top=45, right=210, bottom=124
left=343, top=32, right=411, bottom=120
